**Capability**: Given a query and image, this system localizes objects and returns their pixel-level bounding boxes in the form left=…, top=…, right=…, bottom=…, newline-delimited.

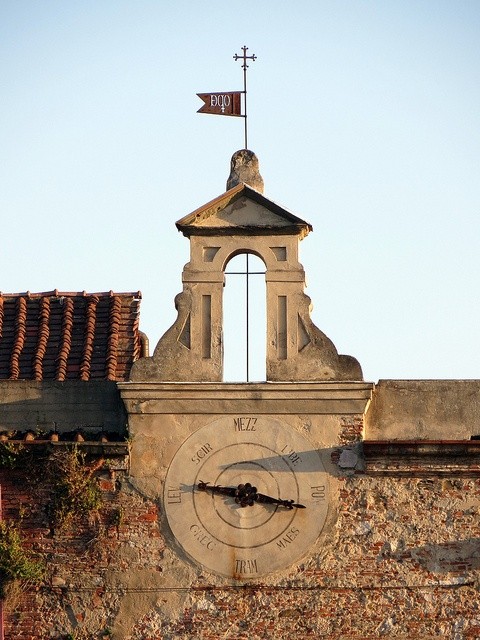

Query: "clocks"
left=163, top=413, right=329, bottom=579
